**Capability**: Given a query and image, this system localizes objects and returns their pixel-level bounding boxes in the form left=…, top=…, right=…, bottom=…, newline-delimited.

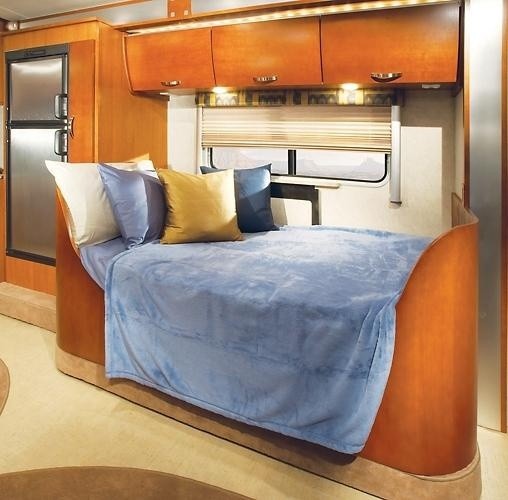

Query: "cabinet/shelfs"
left=320, top=1, right=464, bottom=90
left=211, top=15, right=323, bottom=90
left=122, top=26, right=217, bottom=96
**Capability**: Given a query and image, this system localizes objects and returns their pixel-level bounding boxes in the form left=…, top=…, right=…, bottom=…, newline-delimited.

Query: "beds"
left=54, top=192, right=483, bottom=500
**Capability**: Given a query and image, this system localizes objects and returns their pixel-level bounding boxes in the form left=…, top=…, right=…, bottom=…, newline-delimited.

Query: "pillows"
left=43, top=152, right=151, bottom=250
left=200, top=163, right=280, bottom=234
left=155, top=167, right=247, bottom=244
left=98, top=161, right=167, bottom=251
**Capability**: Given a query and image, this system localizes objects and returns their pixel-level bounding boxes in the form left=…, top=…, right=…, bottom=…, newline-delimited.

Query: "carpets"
left=0, top=354, right=13, bottom=421
left=0, top=464, right=258, bottom=500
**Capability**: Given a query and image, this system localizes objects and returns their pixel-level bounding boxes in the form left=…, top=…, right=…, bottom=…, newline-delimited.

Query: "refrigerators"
left=3, top=42, right=68, bottom=269
